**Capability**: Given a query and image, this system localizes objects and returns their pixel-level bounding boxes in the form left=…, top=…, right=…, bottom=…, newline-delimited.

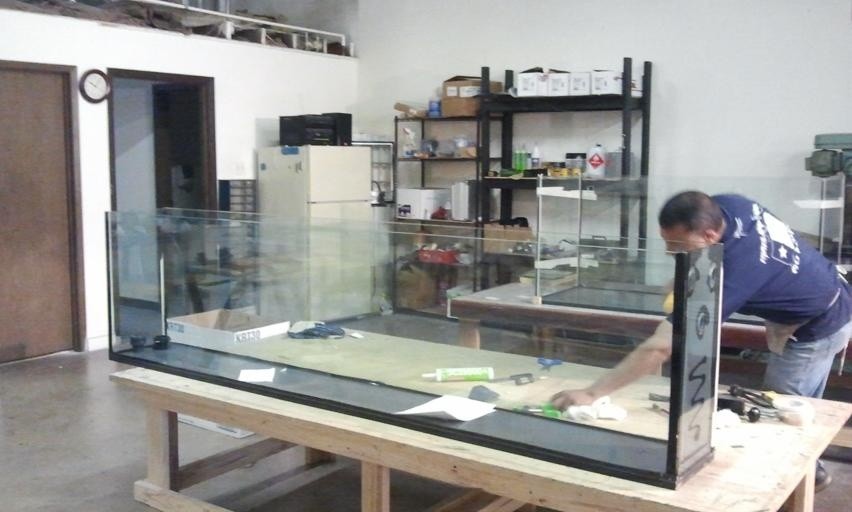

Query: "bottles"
left=427, top=93, right=440, bottom=117
left=585, top=143, right=606, bottom=179
left=514, top=142, right=540, bottom=170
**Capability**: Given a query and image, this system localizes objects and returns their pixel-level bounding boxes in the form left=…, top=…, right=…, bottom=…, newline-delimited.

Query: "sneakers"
left=814, top=465, right=834, bottom=493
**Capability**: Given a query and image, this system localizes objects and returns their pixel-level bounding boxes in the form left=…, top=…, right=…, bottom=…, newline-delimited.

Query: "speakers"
left=322, top=112, right=353, bottom=147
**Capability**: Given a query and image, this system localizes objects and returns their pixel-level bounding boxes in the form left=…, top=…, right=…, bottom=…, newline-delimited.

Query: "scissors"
left=537, top=358, right=563, bottom=372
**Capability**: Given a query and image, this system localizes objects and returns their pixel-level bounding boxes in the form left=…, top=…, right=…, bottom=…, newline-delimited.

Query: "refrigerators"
left=256, top=144, right=372, bottom=323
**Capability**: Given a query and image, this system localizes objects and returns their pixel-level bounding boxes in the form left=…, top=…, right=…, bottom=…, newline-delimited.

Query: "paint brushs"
left=651, top=403, right=670, bottom=416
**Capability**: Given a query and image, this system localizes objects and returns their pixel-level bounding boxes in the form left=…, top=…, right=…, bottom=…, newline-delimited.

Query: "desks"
left=451, top=282, right=769, bottom=359
left=109, top=325, right=852, bottom=512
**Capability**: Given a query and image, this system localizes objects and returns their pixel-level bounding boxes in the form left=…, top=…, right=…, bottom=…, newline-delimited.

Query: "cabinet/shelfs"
left=392, top=57, right=651, bottom=346
left=219, top=180, right=258, bottom=308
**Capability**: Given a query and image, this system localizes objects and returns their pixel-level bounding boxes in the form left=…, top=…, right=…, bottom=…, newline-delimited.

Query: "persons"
left=545, top=190, right=852, bottom=489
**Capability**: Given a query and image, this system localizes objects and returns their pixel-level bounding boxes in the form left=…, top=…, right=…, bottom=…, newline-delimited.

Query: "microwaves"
left=280, top=113, right=350, bottom=145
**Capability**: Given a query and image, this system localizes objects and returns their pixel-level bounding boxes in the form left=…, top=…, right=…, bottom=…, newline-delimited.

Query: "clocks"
left=79, top=70, right=112, bottom=104
